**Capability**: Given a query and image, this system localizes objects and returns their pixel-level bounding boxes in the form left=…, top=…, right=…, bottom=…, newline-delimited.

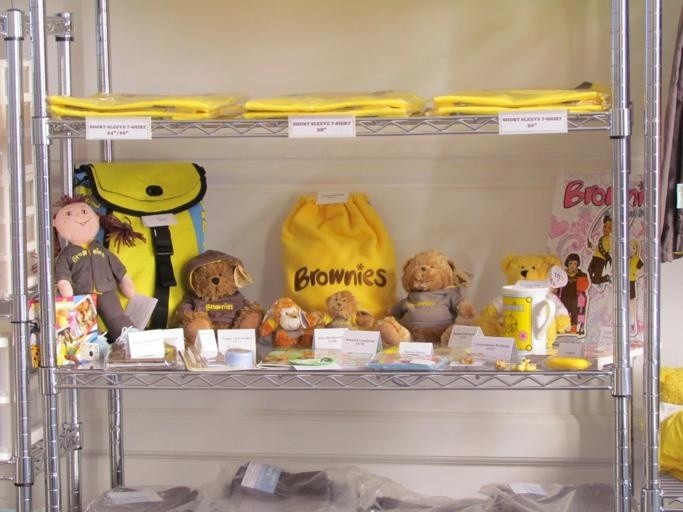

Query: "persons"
left=555, top=251, right=590, bottom=334
left=27, top=313, right=41, bottom=346
left=61, top=330, right=81, bottom=365
left=622, top=238, right=646, bottom=338
left=74, top=297, right=97, bottom=336
left=586, top=215, right=614, bottom=285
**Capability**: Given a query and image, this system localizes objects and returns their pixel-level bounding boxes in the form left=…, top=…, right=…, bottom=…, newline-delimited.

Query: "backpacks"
left=69, top=157, right=209, bottom=334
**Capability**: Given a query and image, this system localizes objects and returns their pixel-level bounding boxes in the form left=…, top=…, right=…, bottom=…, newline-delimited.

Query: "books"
left=544, top=161, right=650, bottom=359
left=22, top=292, right=102, bottom=372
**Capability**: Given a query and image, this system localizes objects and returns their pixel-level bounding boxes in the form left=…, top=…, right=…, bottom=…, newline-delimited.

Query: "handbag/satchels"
left=280, top=189, right=399, bottom=320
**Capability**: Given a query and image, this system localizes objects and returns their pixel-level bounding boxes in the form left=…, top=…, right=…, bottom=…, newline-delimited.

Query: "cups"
left=501, top=285, right=556, bottom=356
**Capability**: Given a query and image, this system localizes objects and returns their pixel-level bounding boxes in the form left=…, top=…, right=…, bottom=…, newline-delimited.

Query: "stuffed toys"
left=50, top=191, right=143, bottom=344
left=474, top=249, right=593, bottom=370
left=258, top=298, right=316, bottom=350
left=375, top=250, right=476, bottom=350
left=305, top=287, right=374, bottom=333
left=168, top=249, right=267, bottom=347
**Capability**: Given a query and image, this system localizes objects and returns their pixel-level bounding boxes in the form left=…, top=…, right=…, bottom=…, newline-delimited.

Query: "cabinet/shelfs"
left=0, top=0, right=683, bottom=511
